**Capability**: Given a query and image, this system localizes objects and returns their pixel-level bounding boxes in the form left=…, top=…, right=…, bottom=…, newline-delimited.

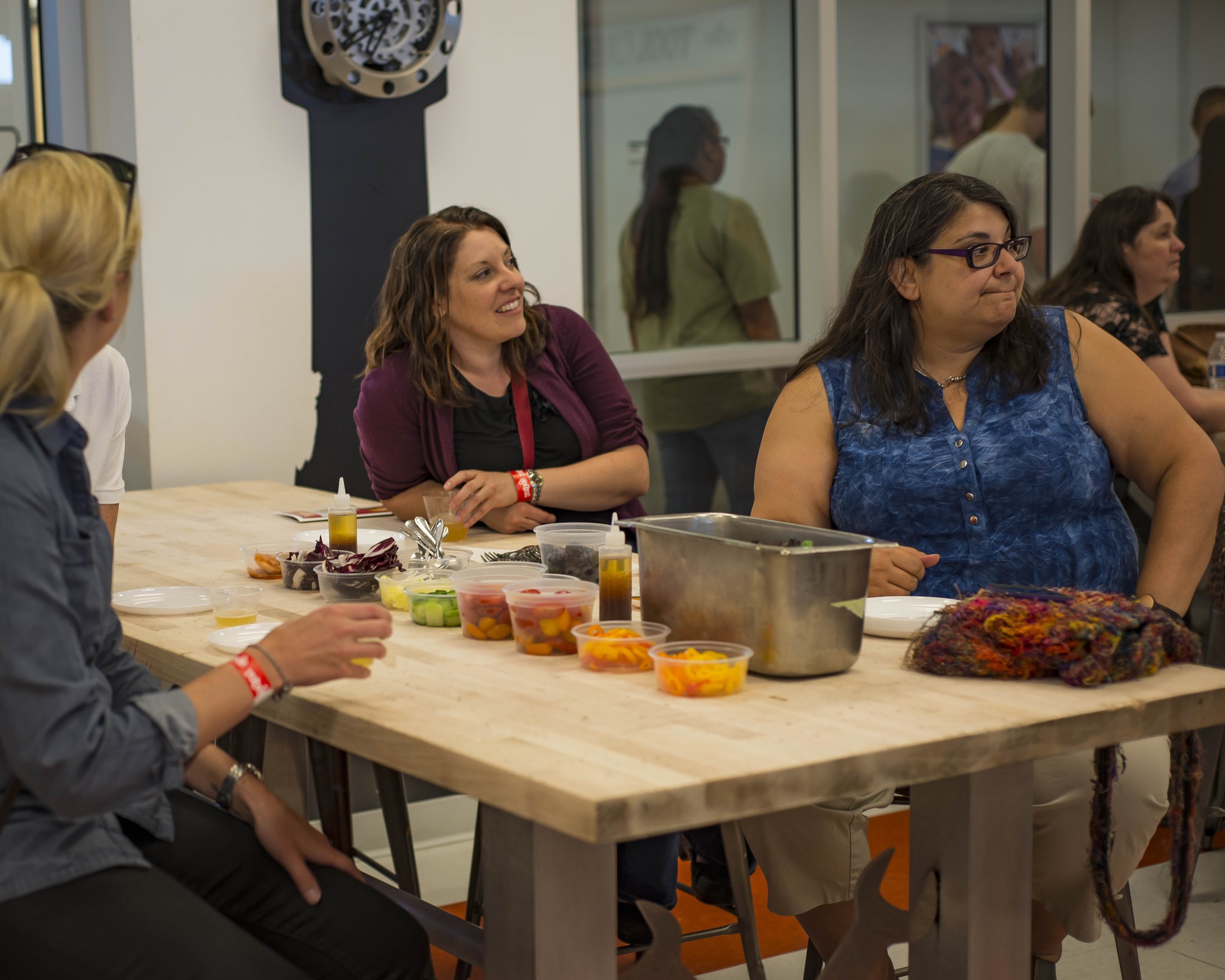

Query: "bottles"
left=599, top=512, right=633, bottom=623
left=328, top=476, right=359, bottom=555
left=1207, top=332, right=1225, bottom=390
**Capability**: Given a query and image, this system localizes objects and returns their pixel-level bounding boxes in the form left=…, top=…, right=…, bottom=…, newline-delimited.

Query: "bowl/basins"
left=395, top=547, right=473, bottom=571
left=502, top=579, right=599, bottom=657
left=375, top=570, right=456, bottom=612
left=572, top=620, right=672, bottom=673
left=276, top=549, right=358, bottom=590
left=240, top=540, right=283, bottom=580
left=533, top=522, right=612, bottom=584
left=648, top=640, right=753, bottom=697
left=313, top=566, right=383, bottom=603
left=453, top=561, right=548, bottom=642
left=403, top=582, right=461, bottom=626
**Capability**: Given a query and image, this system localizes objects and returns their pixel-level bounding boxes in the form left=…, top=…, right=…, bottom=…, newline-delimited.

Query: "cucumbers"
left=411, top=590, right=462, bottom=628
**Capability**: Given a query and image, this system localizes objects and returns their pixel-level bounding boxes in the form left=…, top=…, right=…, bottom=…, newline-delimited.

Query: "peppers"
left=576, top=624, right=660, bottom=671
left=651, top=648, right=747, bottom=696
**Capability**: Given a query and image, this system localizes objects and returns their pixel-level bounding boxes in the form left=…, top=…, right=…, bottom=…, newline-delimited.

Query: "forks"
left=481, top=544, right=542, bottom=564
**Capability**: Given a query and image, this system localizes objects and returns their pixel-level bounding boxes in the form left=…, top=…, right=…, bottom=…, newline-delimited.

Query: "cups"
left=422, top=492, right=472, bottom=543
left=349, top=636, right=379, bottom=669
left=210, top=585, right=263, bottom=627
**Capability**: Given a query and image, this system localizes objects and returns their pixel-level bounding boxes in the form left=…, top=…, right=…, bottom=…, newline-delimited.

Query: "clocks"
left=303, top=1, right=462, bottom=100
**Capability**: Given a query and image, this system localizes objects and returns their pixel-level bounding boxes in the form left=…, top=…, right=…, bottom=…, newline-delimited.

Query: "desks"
left=112, top=484, right=1225, bottom=980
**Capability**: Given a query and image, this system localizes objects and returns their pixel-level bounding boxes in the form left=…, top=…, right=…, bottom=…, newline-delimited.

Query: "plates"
left=209, top=622, right=285, bottom=655
left=292, top=528, right=404, bottom=547
left=862, top=595, right=964, bottom=641
left=110, top=586, right=212, bottom=615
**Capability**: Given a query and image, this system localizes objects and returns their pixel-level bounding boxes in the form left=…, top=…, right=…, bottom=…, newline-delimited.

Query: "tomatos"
left=460, top=584, right=590, bottom=654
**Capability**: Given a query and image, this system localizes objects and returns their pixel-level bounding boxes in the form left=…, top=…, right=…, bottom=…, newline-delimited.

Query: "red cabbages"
left=283, top=534, right=407, bottom=597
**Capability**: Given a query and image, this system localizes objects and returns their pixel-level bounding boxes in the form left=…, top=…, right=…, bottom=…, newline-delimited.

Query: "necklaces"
left=907, top=359, right=967, bottom=389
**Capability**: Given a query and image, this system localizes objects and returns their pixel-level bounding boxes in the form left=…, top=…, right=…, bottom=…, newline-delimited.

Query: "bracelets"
left=508, top=471, right=532, bottom=503
left=248, top=644, right=292, bottom=702
left=1129, top=594, right=1182, bottom=621
left=228, top=652, right=274, bottom=706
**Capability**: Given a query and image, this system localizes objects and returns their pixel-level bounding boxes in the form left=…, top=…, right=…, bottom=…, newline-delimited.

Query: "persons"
left=614, top=103, right=784, bottom=517
left=353, top=204, right=756, bottom=949
left=738, top=171, right=1225, bottom=980
left=940, top=66, right=1225, bottom=312
left=0, top=143, right=434, bottom=980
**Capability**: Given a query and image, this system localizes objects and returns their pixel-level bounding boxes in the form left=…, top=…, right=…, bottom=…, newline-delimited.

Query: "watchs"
left=215, top=761, right=263, bottom=818
left=526, top=469, right=544, bottom=504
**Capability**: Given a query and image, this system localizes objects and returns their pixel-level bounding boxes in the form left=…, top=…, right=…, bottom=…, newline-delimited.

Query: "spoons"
left=400, top=516, right=461, bottom=571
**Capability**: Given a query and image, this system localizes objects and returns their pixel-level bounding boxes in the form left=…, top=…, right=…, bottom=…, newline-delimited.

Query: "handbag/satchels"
left=903, top=584, right=1201, bottom=690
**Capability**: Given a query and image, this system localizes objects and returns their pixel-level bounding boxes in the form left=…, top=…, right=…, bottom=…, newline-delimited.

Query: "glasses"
left=926, top=235, right=1032, bottom=269
left=4, top=143, right=137, bottom=239
left=711, top=135, right=730, bottom=148
